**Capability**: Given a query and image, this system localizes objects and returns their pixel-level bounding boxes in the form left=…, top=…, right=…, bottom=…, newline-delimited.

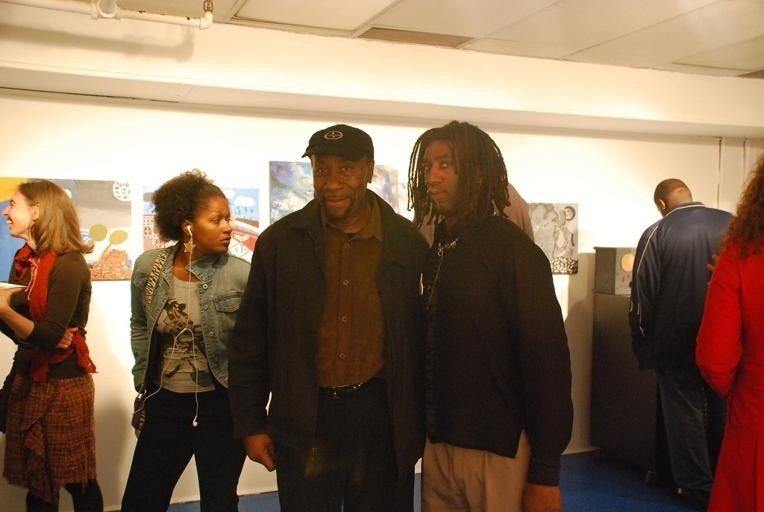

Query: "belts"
left=319, top=363, right=387, bottom=397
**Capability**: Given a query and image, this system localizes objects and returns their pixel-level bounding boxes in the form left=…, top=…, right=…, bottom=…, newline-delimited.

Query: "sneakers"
left=672, top=487, right=709, bottom=512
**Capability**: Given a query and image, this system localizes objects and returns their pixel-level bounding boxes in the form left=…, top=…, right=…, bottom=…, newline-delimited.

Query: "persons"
left=629, top=178, right=739, bottom=512
left=413, top=183, right=533, bottom=251
left=694, top=153, right=764, bottom=512
left=119, top=169, right=253, bottom=512
left=407, top=121, right=574, bottom=512
left=228, top=125, right=430, bottom=512
left=0, top=180, right=103, bottom=512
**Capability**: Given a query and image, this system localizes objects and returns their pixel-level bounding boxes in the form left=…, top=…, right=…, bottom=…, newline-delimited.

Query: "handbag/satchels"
left=128, top=388, right=147, bottom=438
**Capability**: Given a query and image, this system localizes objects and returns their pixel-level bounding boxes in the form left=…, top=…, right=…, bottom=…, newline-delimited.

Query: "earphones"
left=192, top=415, right=199, bottom=427
left=186, top=225, right=193, bottom=235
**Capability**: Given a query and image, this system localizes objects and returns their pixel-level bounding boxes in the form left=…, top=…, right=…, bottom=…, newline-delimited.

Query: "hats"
left=300, top=121, right=373, bottom=165
